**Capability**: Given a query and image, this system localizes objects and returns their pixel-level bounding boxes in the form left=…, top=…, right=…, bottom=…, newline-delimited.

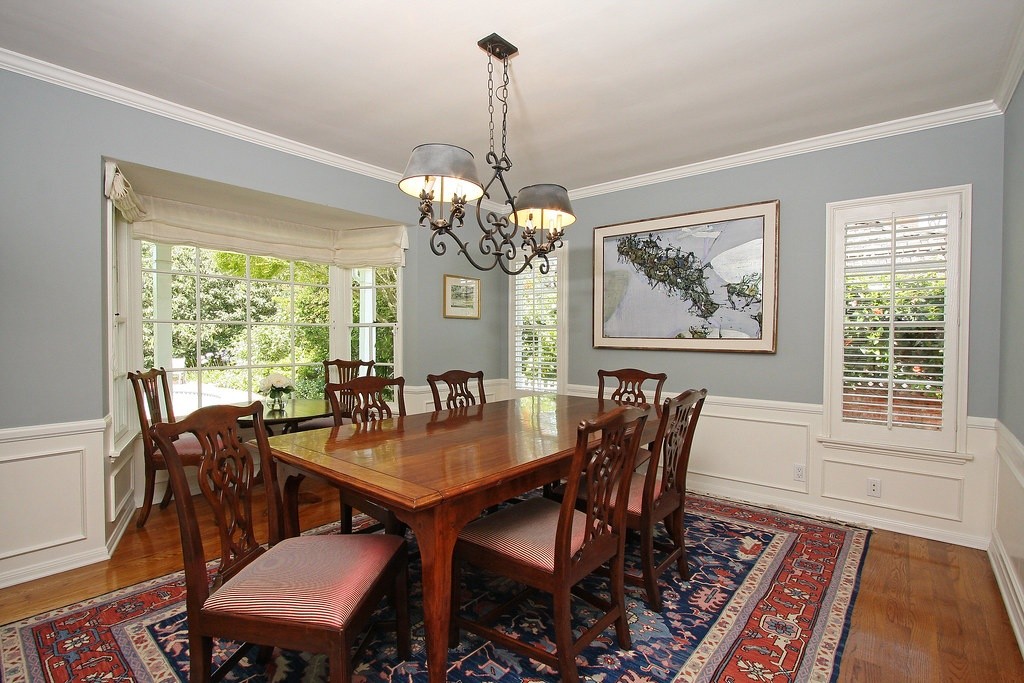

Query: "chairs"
left=447, top=403, right=650, bottom=683
left=543, top=369, right=667, bottom=501
left=554, top=388, right=708, bottom=613
left=149, top=401, right=409, bottom=683
left=427, top=370, right=526, bottom=514
left=289, top=358, right=375, bottom=432
left=323, top=376, right=421, bottom=563
left=127, top=367, right=227, bottom=527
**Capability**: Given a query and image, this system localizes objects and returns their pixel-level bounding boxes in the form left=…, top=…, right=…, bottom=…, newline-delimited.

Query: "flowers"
left=257, top=374, right=295, bottom=409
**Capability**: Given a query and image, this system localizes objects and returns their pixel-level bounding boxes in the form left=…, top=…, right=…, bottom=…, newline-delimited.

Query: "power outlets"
left=867, top=478, right=881, bottom=498
left=794, top=464, right=807, bottom=481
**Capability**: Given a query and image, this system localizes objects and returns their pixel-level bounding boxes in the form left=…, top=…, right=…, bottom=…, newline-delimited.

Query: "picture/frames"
left=443, top=274, right=481, bottom=319
left=592, top=199, right=780, bottom=354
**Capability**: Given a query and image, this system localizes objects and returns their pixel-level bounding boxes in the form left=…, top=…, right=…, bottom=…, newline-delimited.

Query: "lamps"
left=399, top=34, right=575, bottom=276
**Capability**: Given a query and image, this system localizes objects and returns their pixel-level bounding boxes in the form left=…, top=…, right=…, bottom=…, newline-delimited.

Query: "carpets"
left=0, top=480, right=876, bottom=683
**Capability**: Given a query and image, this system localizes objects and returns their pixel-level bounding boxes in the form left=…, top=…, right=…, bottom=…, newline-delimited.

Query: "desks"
left=243, top=391, right=695, bottom=682
left=229, top=400, right=347, bottom=515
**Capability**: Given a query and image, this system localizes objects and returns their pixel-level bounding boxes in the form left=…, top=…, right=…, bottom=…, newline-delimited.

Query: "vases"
left=266, top=392, right=287, bottom=409
